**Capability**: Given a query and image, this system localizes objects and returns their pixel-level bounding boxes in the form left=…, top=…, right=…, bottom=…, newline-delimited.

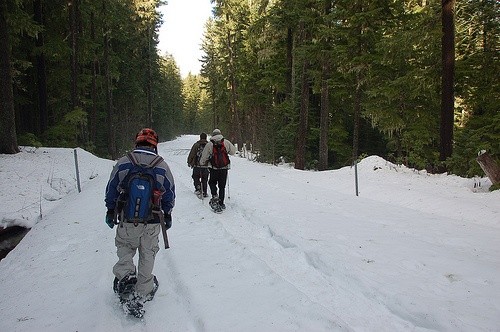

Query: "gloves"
left=163, top=213, right=171, bottom=230
left=106, top=210, right=117, bottom=229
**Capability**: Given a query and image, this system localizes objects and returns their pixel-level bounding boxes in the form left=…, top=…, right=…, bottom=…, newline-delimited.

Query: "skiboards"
left=194, top=189, right=208, bottom=200
left=209, top=192, right=226, bottom=214
left=113, top=270, right=159, bottom=318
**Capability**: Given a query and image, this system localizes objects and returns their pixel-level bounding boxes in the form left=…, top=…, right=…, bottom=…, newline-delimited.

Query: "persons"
left=199, top=129, right=235, bottom=214
left=187, top=133, right=209, bottom=199
left=104, top=128, right=175, bottom=320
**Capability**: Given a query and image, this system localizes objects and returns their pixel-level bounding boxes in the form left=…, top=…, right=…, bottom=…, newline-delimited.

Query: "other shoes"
left=196, top=184, right=201, bottom=194
left=212, top=195, right=220, bottom=205
left=118, top=274, right=137, bottom=295
left=145, top=276, right=159, bottom=302
left=203, top=192, right=208, bottom=197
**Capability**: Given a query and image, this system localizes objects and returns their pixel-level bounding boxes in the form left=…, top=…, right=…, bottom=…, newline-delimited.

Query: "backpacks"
left=118, top=151, right=164, bottom=227
left=208, top=138, right=230, bottom=170
left=195, top=141, right=209, bottom=165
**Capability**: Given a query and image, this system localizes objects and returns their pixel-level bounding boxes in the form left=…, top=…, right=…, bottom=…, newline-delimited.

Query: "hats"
left=200, top=132, right=207, bottom=140
left=134, top=127, right=159, bottom=151
left=212, top=129, right=222, bottom=137
